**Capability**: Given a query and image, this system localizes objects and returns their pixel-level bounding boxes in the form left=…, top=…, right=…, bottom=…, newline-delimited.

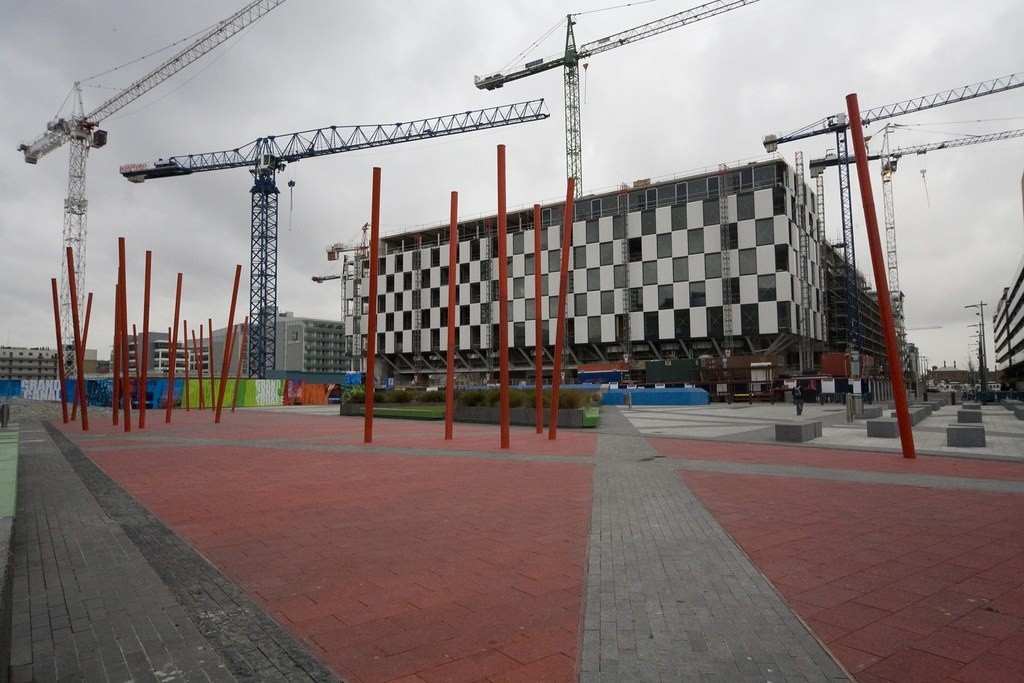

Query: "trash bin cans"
left=909, top=390, right=915, bottom=400
left=851, top=394, right=864, bottom=414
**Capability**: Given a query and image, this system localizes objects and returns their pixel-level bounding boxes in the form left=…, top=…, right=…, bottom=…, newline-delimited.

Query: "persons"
left=792, top=386, right=804, bottom=415
